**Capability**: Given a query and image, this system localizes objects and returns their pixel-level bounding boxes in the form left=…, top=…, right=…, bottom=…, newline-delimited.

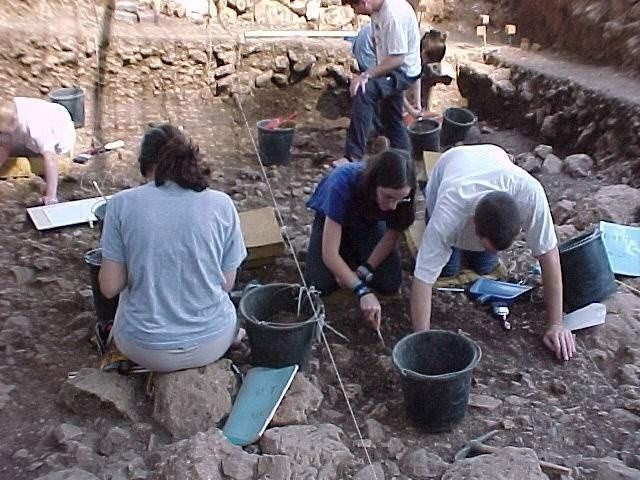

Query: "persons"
left=411, top=143, right=576, bottom=364
left=97, top=124, right=248, bottom=372
left=302, top=149, right=416, bottom=329
left=0, top=93, right=77, bottom=206
left=354, top=24, right=447, bottom=118
left=332, top=0, right=422, bottom=168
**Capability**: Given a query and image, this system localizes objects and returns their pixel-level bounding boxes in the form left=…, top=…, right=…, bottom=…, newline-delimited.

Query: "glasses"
left=376, top=186, right=412, bottom=204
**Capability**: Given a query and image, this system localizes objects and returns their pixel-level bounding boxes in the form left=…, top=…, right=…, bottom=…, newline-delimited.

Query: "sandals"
left=331, top=156, right=361, bottom=168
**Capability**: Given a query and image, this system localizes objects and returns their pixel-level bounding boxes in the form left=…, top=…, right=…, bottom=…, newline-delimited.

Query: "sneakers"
left=94, top=320, right=131, bottom=370
left=234, top=329, right=246, bottom=347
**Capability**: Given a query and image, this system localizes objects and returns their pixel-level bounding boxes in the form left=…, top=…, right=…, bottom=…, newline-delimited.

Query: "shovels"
left=264, top=111, right=297, bottom=129
left=469, top=277, right=536, bottom=303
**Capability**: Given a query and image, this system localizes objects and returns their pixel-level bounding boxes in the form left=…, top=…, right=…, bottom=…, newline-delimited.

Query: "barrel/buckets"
left=390, top=328, right=481, bottom=436
left=91, top=197, right=109, bottom=224
left=50, top=88, right=85, bottom=131
left=540, top=229, right=612, bottom=303
left=406, top=119, right=441, bottom=156
left=256, top=117, right=296, bottom=165
left=237, top=284, right=323, bottom=379
left=84, top=245, right=124, bottom=323
left=441, top=107, right=475, bottom=148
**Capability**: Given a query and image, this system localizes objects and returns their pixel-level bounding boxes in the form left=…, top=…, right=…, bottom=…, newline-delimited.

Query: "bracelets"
left=348, top=280, right=374, bottom=297
left=355, top=260, right=374, bottom=280
